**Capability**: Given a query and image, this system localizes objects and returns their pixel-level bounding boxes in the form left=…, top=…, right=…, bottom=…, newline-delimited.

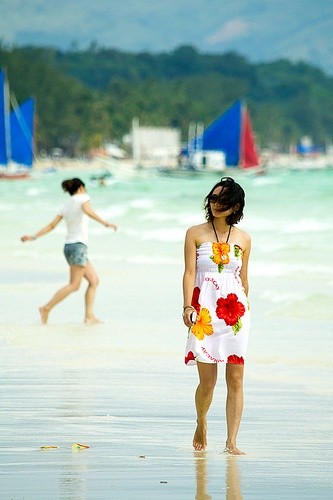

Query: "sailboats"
left=1, top=67, right=35, bottom=179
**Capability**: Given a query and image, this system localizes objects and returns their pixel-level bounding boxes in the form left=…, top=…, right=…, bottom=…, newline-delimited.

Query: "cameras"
left=190, top=311, right=196, bottom=323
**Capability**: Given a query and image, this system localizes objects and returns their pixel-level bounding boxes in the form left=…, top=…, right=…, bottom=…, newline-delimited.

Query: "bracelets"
left=183, top=304, right=194, bottom=310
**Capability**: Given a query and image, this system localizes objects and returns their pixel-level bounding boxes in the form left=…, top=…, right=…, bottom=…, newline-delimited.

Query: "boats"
left=159, top=167, right=223, bottom=179
left=90, top=171, right=117, bottom=188
left=291, top=160, right=326, bottom=171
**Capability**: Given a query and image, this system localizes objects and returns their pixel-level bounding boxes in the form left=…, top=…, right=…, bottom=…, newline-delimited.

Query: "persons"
left=182, top=177, right=253, bottom=455
left=20, top=178, right=116, bottom=325
